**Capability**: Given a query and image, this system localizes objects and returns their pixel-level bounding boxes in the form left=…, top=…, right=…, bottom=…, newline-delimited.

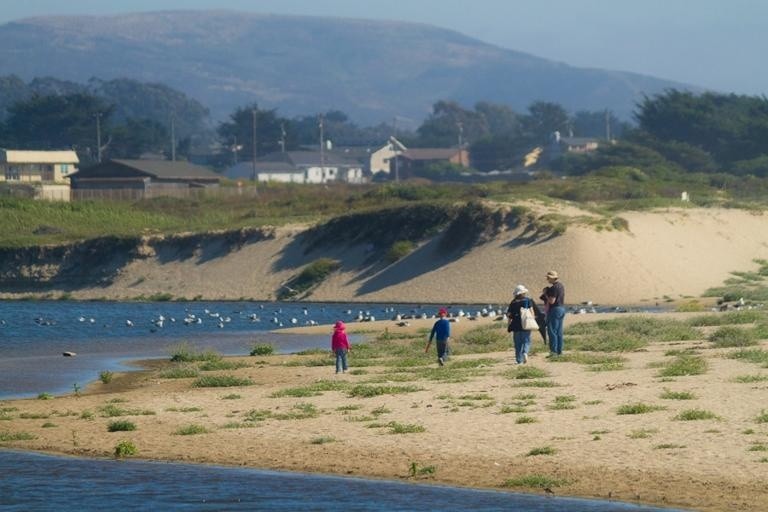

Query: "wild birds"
left=151, top=304, right=510, bottom=327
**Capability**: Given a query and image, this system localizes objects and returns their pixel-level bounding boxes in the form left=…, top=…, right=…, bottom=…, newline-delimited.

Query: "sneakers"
left=523, top=353, right=527, bottom=363
left=439, top=358, right=444, bottom=366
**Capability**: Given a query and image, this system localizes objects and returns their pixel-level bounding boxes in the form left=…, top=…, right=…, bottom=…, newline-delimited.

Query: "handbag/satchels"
left=520, top=307, right=539, bottom=329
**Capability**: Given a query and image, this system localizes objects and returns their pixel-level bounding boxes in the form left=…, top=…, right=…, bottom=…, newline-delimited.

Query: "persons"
left=540, top=285, right=550, bottom=321
left=543, top=269, right=565, bottom=362
left=329, top=320, right=352, bottom=375
left=425, top=307, right=452, bottom=367
left=504, top=283, right=537, bottom=366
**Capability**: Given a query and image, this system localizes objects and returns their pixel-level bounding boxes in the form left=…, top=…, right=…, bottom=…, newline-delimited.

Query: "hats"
left=513, top=285, right=528, bottom=295
left=439, top=308, right=446, bottom=313
left=545, top=271, right=559, bottom=278
left=334, top=321, right=343, bottom=328
